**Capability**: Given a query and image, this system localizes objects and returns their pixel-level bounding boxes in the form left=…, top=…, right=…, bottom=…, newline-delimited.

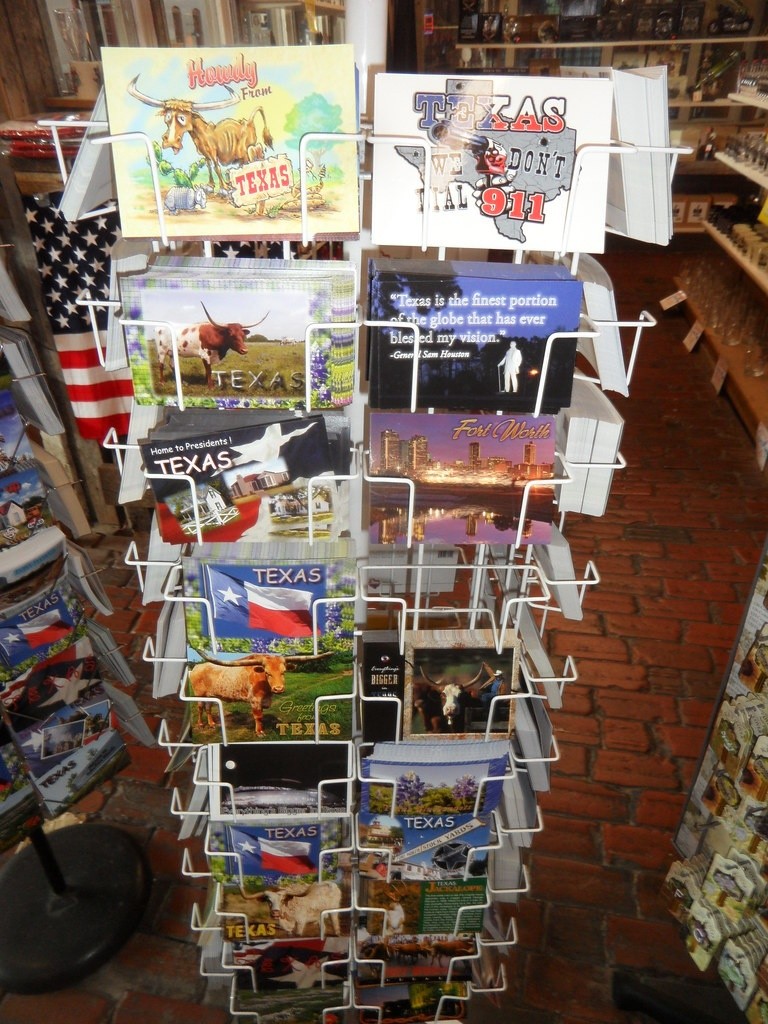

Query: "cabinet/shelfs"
left=385, top=0, right=768, bottom=236
left=667, top=93, right=768, bottom=459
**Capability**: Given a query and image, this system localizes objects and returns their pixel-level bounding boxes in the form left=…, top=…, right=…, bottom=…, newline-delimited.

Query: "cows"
left=413, top=662, right=486, bottom=734
left=152, top=299, right=271, bottom=392
left=360, top=938, right=476, bottom=970
left=189, top=647, right=335, bottom=740
left=238, top=882, right=341, bottom=938
